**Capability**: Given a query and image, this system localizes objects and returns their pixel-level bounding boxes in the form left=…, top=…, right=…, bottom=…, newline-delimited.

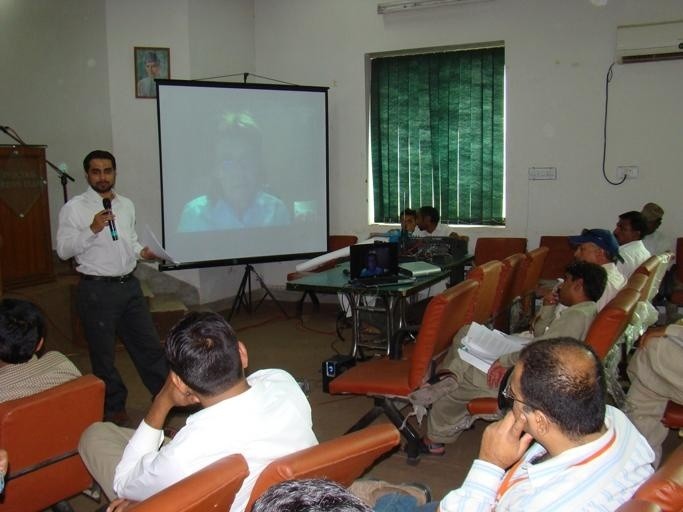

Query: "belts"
left=82, top=274, right=134, bottom=283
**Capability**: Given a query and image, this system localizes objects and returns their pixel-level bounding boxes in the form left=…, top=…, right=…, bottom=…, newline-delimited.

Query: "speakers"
left=321, top=354, right=357, bottom=393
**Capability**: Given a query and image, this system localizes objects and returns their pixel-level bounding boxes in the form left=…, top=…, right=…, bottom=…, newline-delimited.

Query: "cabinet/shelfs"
left=0, top=144, right=55, bottom=294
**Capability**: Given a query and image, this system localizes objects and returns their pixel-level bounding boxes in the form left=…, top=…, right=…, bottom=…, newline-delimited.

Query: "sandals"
left=399, top=437, right=446, bottom=457
left=407, top=371, right=460, bottom=406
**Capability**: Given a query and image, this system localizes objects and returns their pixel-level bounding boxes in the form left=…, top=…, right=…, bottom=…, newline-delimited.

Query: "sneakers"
left=346, top=479, right=432, bottom=506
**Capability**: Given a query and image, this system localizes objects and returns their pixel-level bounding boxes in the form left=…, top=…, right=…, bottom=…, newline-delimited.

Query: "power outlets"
left=616, top=166, right=639, bottom=178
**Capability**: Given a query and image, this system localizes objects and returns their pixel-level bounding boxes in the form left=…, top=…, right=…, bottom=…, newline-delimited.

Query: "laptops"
left=350, top=242, right=404, bottom=287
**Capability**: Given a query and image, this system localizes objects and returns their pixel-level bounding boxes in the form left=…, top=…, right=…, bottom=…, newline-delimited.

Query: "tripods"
left=227, top=266, right=290, bottom=321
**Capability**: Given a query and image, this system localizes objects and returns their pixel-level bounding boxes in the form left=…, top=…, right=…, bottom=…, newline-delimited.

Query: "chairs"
left=242, top=423, right=400, bottom=512
left=619, top=441, right=683, bottom=512
left=0, top=373, right=106, bottom=512
left=121, top=454, right=250, bottom=512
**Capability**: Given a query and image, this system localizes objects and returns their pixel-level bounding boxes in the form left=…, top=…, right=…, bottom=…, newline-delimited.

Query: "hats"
left=640, top=202, right=664, bottom=222
left=144, top=52, right=157, bottom=63
left=570, top=229, right=626, bottom=264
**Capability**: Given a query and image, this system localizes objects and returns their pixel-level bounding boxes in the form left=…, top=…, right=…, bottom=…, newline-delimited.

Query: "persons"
left=0, top=299, right=81, bottom=404
left=359, top=253, right=384, bottom=277
left=77, top=310, right=320, bottom=512
left=251, top=477, right=374, bottom=512
left=398, top=203, right=682, bottom=472
left=177, top=112, right=296, bottom=231
left=56, top=150, right=202, bottom=428
left=347, top=336, right=656, bottom=512
left=137, top=51, right=164, bottom=97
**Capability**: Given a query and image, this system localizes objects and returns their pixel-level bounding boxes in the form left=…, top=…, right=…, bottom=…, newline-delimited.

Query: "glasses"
left=501, top=384, right=544, bottom=413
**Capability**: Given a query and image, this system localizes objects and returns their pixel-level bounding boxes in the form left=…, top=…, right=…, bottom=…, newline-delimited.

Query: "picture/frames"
left=134, top=48, right=170, bottom=98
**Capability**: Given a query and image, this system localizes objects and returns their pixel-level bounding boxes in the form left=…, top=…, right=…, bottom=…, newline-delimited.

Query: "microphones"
left=103, top=198, right=118, bottom=241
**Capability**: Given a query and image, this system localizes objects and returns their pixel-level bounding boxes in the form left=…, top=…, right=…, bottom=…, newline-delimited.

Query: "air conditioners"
left=615, top=21, right=683, bottom=63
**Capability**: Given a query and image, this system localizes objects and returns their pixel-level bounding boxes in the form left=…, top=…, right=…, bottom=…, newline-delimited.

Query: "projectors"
left=398, top=261, right=442, bottom=279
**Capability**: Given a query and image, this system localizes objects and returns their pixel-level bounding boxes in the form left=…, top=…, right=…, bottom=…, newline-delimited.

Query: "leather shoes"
left=105, top=411, right=133, bottom=429
